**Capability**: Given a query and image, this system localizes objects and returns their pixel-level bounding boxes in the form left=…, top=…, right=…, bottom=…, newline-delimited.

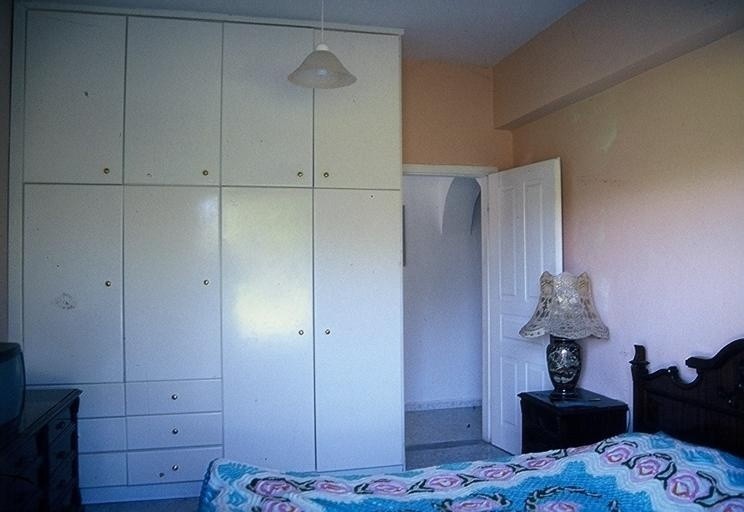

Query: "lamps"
left=287, top=0, right=357, bottom=90
left=518, top=270, right=610, bottom=402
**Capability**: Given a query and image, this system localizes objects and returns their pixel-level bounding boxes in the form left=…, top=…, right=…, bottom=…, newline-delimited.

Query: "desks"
left=1, top=390, right=80, bottom=512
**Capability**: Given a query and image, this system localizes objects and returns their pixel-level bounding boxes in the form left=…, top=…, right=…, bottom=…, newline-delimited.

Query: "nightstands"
left=517, top=387, right=630, bottom=456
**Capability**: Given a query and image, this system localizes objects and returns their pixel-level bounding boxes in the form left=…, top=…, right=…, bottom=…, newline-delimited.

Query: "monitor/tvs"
left=0, top=342, right=26, bottom=448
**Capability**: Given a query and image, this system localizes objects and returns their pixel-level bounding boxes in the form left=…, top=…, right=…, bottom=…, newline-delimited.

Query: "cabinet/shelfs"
left=79, top=452, right=129, bottom=488
left=219, top=21, right=314, bottom=186
left=124, top=186, right=221, bottom=379
left=79, top=418, right=126, bottom=454
left=220, top=187, right=316, bottom=476
left=22, top=184, right=125, bottom=386
left=25, top=383, right=126, bottom=419
left=125, top=378, right=223, bottom=416
left=126, top=412, right=223, bottom=452
left=128, top=446, right=223, bottom=486
left=314, top=30, right=402, bottom=187
left=22, top=8, right=126, bottom=186
left=316, top=190, right=405, bottom=471
left=122, top=13, right=222, bottom=186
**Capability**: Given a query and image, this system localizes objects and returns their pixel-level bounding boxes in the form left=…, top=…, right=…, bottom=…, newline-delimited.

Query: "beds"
left=198, top=335, right=744, bottom=512
